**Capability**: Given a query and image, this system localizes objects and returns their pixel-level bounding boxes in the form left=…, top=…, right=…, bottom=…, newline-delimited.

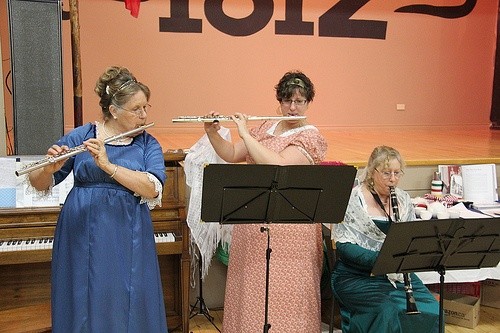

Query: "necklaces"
left=102, top=121, right=129, bottom=142
left=372, top=191, right=393, bottom=222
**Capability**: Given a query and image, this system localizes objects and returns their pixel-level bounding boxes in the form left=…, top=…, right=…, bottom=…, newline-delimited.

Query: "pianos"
left=0, top=149, right=189, bottom=333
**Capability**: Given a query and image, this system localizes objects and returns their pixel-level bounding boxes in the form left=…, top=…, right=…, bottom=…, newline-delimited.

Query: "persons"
left=330, top=145, right=445, bottom=333
left=204, top=71, right=328, bottom=333
left=29, top=65, right=166, bottom=333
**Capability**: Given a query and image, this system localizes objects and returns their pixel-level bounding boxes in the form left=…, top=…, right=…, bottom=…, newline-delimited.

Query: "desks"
left=414, top=207, right=500, bottom=289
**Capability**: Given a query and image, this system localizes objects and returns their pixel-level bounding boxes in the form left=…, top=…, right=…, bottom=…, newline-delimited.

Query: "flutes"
left=15, top=122, right=155, bottom=176
left=391, top=186, right=421, bottom=316
left=172, top=116, right=308, bottom=123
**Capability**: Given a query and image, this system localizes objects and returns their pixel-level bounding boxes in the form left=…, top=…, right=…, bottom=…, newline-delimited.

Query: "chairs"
left=322, top=226, right=335, bottom=333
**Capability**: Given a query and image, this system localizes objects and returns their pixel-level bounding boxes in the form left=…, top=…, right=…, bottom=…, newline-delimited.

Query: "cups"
left=414, top=207, right=426, bottom=217
left=437, top=211, right=449, bottom=219
left=420, top=211, right=433, bottom=220
left=449, top=210, right=460, bottom=219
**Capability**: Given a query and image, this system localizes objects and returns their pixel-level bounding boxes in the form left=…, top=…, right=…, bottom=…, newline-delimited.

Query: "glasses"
left=375, top=167, right=405, bottom=181
left=111, top=102, right=152, bottom=118
left=282, top=96, right=308, bottom=107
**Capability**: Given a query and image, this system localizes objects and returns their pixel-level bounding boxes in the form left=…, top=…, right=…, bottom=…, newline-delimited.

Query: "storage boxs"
left=443, top=292, right=481, bottom=329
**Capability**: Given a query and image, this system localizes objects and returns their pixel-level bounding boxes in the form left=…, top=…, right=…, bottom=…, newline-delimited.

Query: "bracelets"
left=110, top=164, right=117, bottom=178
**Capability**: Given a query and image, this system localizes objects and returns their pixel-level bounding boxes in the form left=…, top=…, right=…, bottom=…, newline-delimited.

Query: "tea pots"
left=426, top=200, right=447, bottom=218
